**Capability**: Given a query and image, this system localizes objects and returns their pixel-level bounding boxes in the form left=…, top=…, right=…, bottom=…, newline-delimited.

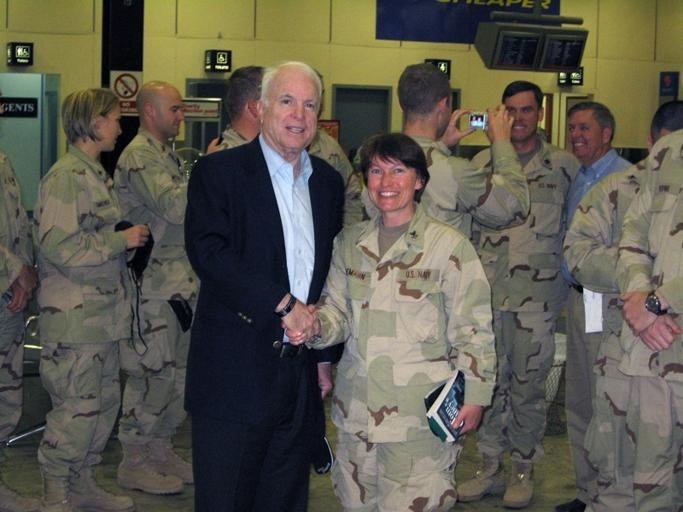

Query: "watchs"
left=644, top=289, right=668, bottom=316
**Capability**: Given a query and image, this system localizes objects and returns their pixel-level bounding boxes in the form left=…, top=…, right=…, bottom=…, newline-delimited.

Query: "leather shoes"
left=555, top=498, right=586, bottom=512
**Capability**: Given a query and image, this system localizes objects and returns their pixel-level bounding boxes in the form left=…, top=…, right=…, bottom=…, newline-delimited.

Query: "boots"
left=456, top=454, right=507, bottom=502
left=69, top=467, right=134, bottom=511
left=116, top=441, right=183, bottom=495
left=502, top=457, right=535, bottom=507
left=149, top=434, right=193, bottom=485
left=0, top=475, right=41, bottom=512
left=41, top=468, right=77, bottom=512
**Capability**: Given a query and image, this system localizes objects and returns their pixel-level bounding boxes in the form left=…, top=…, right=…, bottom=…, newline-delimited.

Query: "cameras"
left=469, top=112, right=489, bottom=130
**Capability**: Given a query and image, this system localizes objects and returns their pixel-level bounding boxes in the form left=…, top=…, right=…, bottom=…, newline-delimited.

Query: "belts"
left=278, top=342, right=305, bottom=358
left=571, top=282, right=584, bottom=293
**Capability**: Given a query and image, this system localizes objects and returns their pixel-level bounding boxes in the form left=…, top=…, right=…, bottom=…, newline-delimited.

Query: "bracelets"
left=274, top=293, right=297, bottom=318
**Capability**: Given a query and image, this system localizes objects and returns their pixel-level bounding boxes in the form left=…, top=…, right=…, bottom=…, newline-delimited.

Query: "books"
left=424, top=368, right=468, bottom=444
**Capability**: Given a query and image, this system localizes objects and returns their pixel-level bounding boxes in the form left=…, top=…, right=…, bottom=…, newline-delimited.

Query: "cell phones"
left=215, top=132, right=226, bottom=147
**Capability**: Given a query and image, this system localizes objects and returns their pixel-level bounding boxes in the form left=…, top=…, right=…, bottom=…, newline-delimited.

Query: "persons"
left=0, top=148, right=40, bottom=512
left=305, top=74, right=363, bottom=474
left=280, top=131, right=497, bottom=512
left=112, top=78, right=224, bottom=494
left=454, top=81, right=580, bottom=508
left=30, top=87, right=149, bottom=512
left=562, top=100, right=682, bottom=511
left=184, top=61, right=346, bottom=512
left=353, top=62, right=531, bottom=242
left=555, top=101, right=634, bottom=512
left=617, top=126, right=683, bottom=512
left=218, top=64, right=263, bottom=149
